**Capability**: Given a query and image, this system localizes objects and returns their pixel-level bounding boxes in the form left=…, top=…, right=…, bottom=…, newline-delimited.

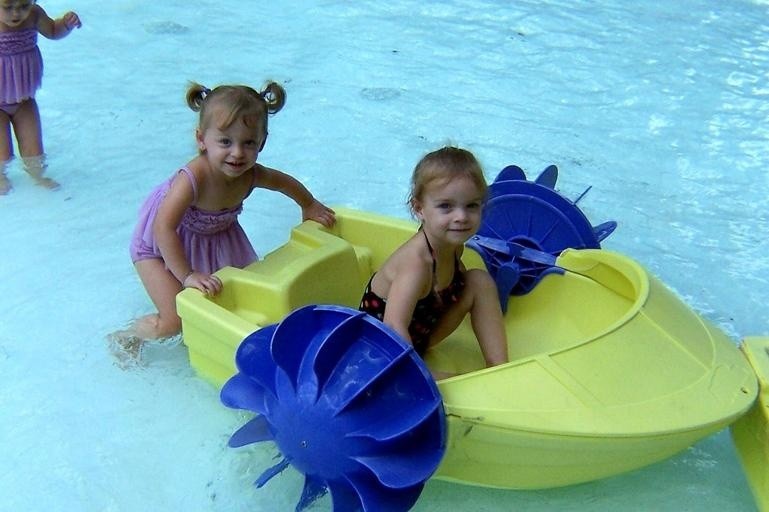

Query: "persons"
left=0, top=1, right=82, bottom=198
left=357, top=146, right=509, bottom=381
left=105, top=79, right=336, bottom=370
